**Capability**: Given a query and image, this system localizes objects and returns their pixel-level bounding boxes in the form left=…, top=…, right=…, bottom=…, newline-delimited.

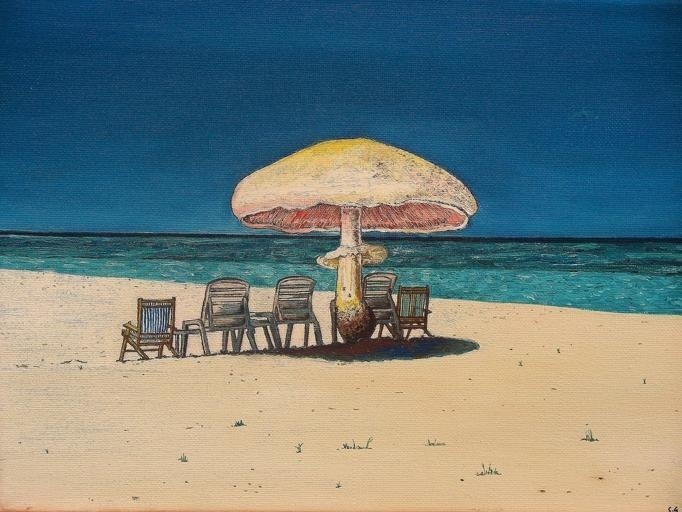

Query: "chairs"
left=116, top=272, right=436, bottom=359
